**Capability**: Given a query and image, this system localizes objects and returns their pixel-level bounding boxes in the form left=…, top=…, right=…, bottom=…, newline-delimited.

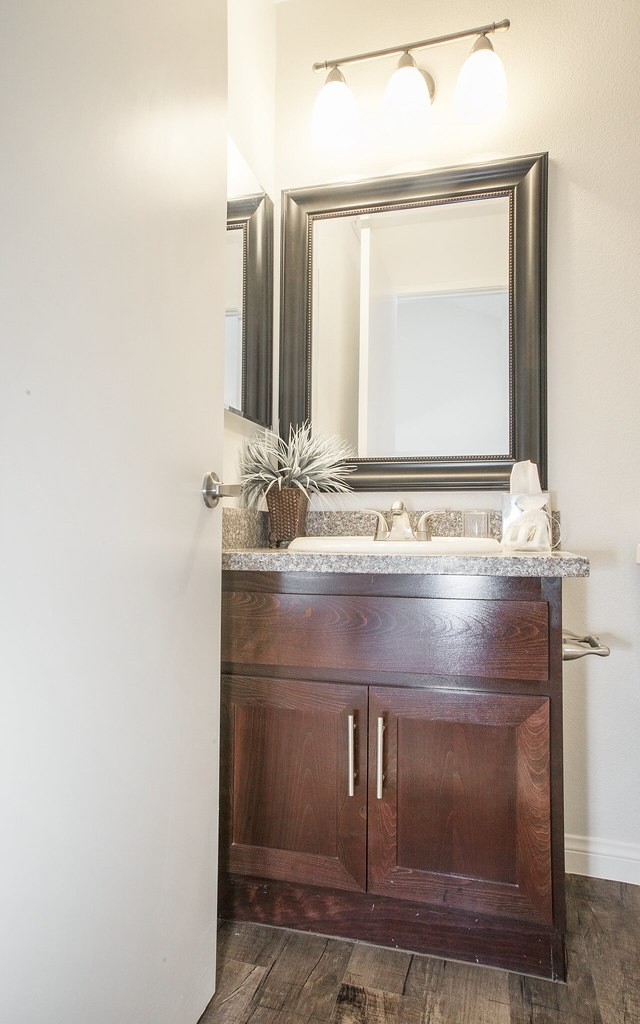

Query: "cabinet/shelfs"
left=220, top=570, right=564, bottom=983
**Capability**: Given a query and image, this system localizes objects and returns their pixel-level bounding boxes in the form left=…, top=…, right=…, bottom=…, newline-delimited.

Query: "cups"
left=463, top=510, right=489, bottom=537
left=502, top=492, right=552, bottom=551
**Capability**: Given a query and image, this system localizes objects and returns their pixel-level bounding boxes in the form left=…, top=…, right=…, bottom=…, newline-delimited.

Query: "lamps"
left=394, top=49, right=417, bottom=74
left=469, top=31, right=494, bottom=56
left=324, top=64, right=346, bottom=86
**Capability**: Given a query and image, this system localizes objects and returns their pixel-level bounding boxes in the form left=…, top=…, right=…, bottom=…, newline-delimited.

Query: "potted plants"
left=238, top=419, right=357, bottom=542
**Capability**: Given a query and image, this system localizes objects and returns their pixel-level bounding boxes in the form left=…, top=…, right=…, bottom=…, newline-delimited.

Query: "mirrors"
left=280, top=151, right=548, bottom=493
left=225, top=190, right=274, bottom=429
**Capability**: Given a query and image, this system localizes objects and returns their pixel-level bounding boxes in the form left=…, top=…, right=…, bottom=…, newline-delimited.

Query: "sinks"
left=287, top=535, right=501, bottom=554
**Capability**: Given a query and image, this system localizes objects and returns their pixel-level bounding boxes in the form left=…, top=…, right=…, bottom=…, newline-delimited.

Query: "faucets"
left=358, top=500, right=448, bottom=542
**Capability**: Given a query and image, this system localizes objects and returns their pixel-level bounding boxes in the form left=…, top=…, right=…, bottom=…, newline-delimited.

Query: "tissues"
left=501, top=459, right=553, bottom=551
